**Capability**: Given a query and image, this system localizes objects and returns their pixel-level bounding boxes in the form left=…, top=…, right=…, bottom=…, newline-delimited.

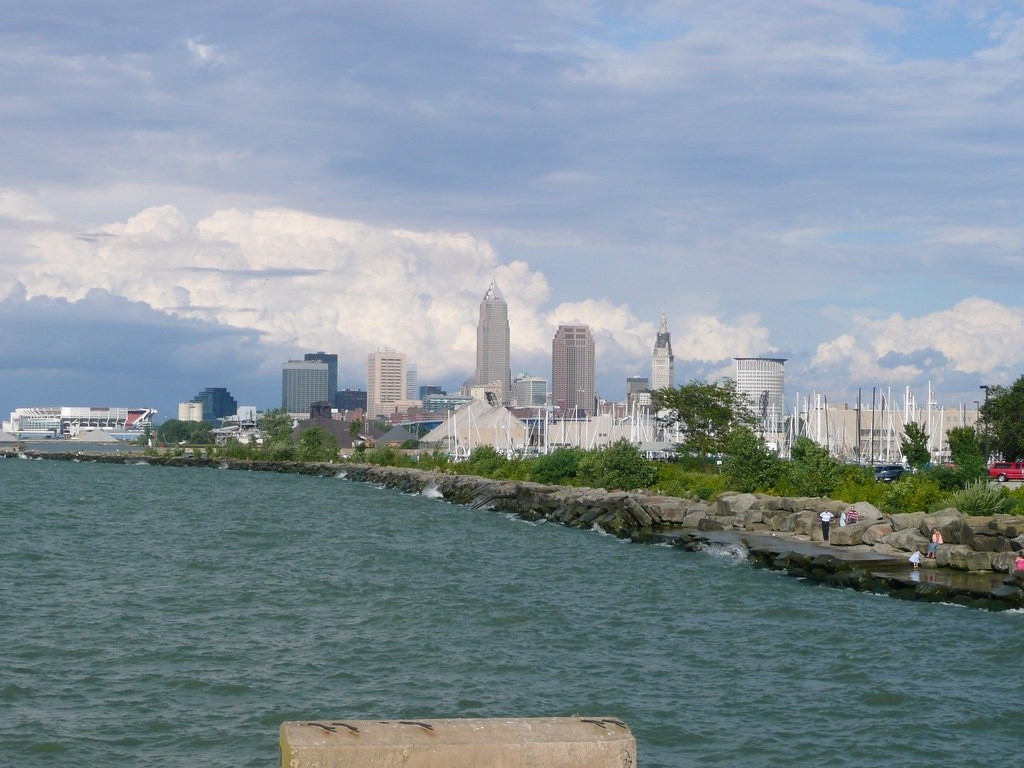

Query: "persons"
left=1015, top=551, right=1024, bottom=571
left=846, top=505, right=860, bottom=524
left=839, top=509, right=847, bottom=526
left=820, top=508, right=834, bottom=541
left=926, top=527, right=943, bottom=558
left=908, top=548, right=924, bottom=568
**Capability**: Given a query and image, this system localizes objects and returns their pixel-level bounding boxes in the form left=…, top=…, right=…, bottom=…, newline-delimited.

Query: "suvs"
left=990, top=461, right=1024, bottom=483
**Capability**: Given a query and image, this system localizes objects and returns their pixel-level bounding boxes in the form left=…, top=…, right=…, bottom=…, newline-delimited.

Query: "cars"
left=871, top=465, right=906, bottom=483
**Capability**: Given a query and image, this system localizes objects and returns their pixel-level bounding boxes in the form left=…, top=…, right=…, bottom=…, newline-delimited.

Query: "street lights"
left=973, top=401, right=981, bottom=444
left=979, top=385, right=988, bottom=482
